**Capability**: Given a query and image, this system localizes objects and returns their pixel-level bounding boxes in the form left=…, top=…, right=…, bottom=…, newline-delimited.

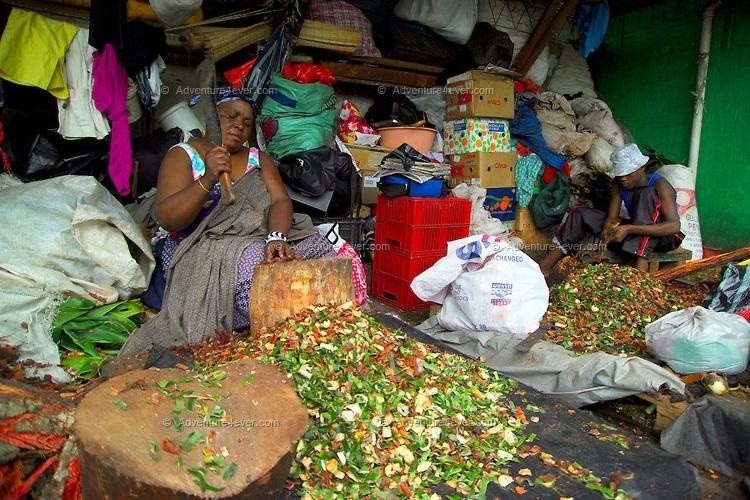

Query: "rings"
left=273, top=242, right=283, bottom=251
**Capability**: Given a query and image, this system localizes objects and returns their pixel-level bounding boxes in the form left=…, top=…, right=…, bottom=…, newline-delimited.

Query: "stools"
left=643, top=248, right=693, bottom=274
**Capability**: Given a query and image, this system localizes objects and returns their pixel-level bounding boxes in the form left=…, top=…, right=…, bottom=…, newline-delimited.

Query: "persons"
left=539, top=143, right=687, bottom=278
left=153, top=90, right=336, bottom=330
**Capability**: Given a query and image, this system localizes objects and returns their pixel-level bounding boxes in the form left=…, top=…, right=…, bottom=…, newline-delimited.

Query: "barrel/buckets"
left=158, top=102, right=204, bottom=138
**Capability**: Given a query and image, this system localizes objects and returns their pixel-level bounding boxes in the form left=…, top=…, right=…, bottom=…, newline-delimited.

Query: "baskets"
left=373, top=217, right=470, bottom=254
left=311, top=217, right=362, bottom=260
left=371, top=241, right=450, bottom=283
left=369, top=268, right=431, bottom=313
left=375, top=192, right=472, bottom=227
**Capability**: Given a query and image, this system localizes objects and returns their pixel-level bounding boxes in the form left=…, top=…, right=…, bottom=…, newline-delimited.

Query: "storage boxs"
left=369, top=71, right=518, bottom=309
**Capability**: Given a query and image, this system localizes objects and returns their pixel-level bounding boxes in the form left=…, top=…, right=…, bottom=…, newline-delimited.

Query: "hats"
left=605, top=141, right=651, bottom=178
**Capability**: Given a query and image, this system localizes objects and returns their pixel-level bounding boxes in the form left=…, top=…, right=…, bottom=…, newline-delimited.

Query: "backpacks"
left=277, top=145, right=357, bottom=217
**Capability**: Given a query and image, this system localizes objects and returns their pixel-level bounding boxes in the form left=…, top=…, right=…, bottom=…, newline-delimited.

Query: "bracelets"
left=197, top=177, right=215, bottom=194
left=264, top=230, right=287, bottom=244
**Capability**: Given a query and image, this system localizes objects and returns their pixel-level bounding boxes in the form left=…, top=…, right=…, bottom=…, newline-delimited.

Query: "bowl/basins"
left=384, top=174, right=444, bottom=198
left=376, top=125, right=439, bottom=158
